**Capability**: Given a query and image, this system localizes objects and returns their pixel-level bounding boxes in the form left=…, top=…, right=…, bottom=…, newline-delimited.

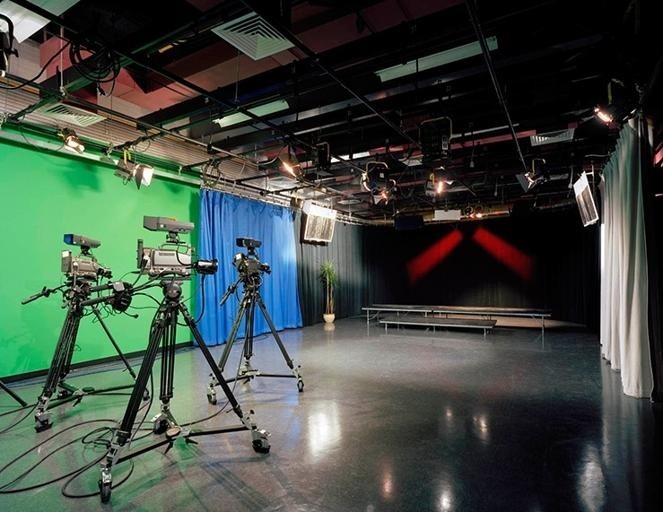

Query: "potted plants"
left=317, top=257, right=342, bottom=324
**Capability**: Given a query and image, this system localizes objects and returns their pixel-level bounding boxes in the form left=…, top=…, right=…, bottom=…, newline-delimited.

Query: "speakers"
left=392, top=214, right=425, bottom=232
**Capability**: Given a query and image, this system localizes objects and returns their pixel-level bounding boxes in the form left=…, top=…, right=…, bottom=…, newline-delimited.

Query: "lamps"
left=365, top=166, right=390, bottom=190
left=514, top=168, right=545, bottom=193
left=56, top=125, right=156, bottom=191
left=593, top=78, right=625, bottom=129
